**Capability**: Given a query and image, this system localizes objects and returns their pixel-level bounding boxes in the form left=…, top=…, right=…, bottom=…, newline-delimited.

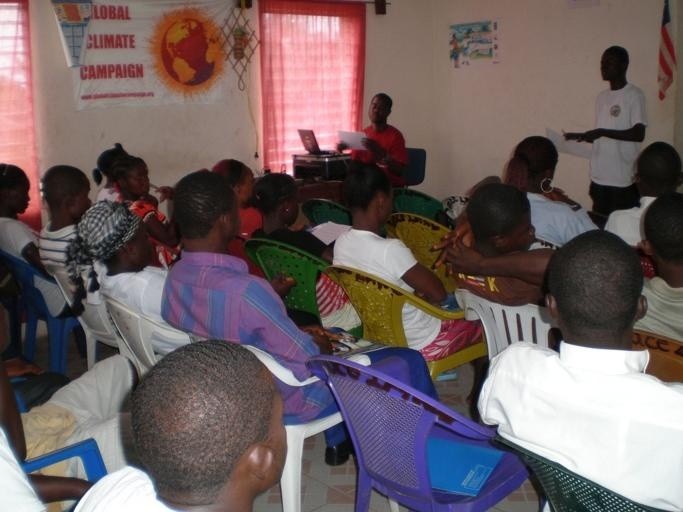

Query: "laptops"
left=298, top=130, right=339, bottom=154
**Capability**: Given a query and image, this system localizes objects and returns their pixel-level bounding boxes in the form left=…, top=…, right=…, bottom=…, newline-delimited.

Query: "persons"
left=337, top=92, right=413, bottom=186
left=1, top=136, right=682, bottom=512
left=564, top=45, right=646, bottom=214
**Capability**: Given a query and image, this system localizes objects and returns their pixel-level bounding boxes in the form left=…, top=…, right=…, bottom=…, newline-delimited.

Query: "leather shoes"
left=325, top=440, right=353, bottom=466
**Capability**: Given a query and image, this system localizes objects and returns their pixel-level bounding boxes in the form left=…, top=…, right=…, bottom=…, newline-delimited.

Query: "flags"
left=656, top=1, right=677, bottom=99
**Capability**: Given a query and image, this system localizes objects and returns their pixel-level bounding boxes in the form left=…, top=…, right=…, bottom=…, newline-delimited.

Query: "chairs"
left=403, top=148, right=426, bottom=188
